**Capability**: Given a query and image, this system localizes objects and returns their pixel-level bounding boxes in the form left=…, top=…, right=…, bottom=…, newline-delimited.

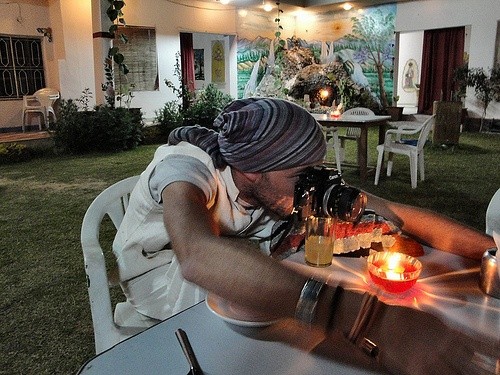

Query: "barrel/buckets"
left=432, top=101, right=463, bottom=145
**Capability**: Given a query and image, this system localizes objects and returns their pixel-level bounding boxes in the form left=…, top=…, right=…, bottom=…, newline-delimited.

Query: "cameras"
left=294, top=165, right=368, bottom=222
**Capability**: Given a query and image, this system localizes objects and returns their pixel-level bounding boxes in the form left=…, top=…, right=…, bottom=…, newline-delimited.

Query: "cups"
left=305, top=210, right=335, bottom=268
left=479, top=247, right=500, bottom=299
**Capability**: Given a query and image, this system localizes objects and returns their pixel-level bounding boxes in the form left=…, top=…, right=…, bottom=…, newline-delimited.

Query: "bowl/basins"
left=367, top=251, right=422, bottom=295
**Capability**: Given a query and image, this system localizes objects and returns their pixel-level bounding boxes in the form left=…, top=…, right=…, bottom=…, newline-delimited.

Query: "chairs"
left=80, top=175, right=142, bottom=355
left=373, top=114, right=438, bottom=191
left=21, top=88, right=60, bottom=134
left=327, top=107, right=375, bottom=161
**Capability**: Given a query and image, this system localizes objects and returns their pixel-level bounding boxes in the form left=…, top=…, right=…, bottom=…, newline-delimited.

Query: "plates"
left=205, top=290, right=282, bottom=328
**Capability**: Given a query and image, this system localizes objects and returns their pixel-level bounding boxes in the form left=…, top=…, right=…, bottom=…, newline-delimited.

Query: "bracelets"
left=347, top=291, right=384, bottom=357
left=323, top=285, right=345, bottom=331
left=295, top=278, right=327, bottom=324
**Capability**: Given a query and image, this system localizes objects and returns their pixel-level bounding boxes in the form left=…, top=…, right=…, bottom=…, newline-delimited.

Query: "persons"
left=114, top=96, right=128, bottom=110
left=112, top=97, right=500, bottom=375
left=257, top=55, right=270, bottom=86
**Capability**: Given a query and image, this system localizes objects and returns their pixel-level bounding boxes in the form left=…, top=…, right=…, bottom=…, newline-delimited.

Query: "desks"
left=76, top=234, right=500, bottom=375
left=310, top=112, right=391, bottom=188
